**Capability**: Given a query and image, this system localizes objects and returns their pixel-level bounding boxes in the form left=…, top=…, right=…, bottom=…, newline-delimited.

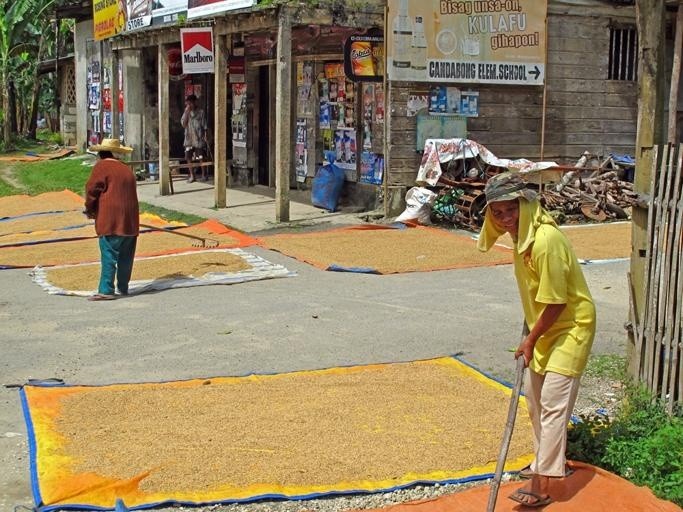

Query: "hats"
left=89, top=138, right=133, bottom=153
left=479, top=170, right=538, bottom=214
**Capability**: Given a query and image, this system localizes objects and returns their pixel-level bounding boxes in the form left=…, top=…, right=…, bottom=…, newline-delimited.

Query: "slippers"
left=88, top=294, right=116, bottom=301
left=518, top=467, right=574, bottom=479
left=508, top=488, right=552, bottom=508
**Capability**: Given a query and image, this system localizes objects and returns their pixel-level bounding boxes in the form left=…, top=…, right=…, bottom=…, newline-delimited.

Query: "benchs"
left=127, top=157, right=236, bottom=195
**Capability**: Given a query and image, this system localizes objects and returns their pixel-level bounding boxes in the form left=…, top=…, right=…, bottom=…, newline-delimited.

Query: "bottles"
left=390, top=0, right=428, bottom=78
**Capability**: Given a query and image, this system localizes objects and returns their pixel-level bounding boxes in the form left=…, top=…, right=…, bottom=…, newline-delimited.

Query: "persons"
left=179, top=93, right=210, bottom=183
left=81, top=138, right=139, bottom=302
left=476, top=170, right=596, bottom=508
